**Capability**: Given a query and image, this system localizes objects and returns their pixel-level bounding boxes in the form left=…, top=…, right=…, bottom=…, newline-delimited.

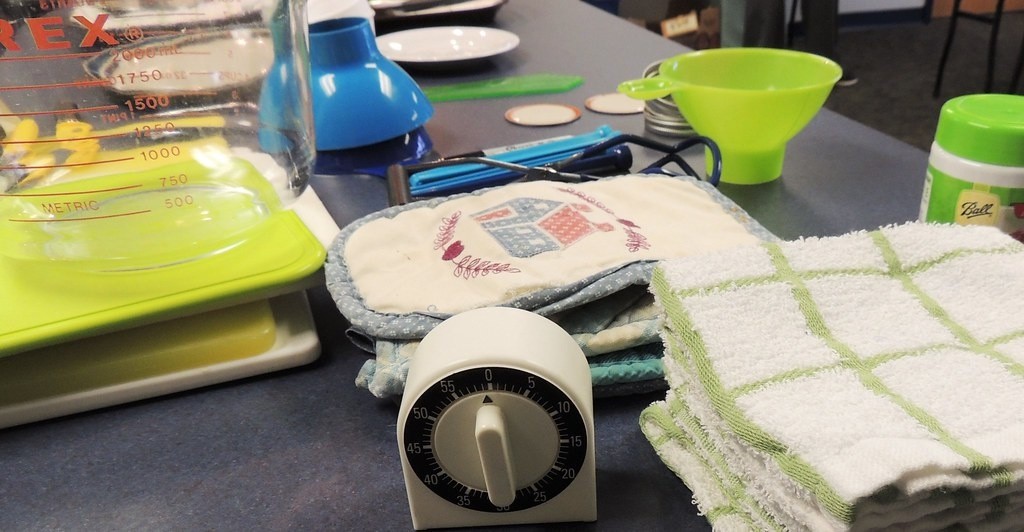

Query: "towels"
left=639, top=218, right=1024, bottom=532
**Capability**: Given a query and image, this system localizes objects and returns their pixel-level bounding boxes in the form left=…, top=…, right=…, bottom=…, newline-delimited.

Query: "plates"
left=66, top=0, right=275, bottom=33
left=373, top=25, right=521, bottom=70
left=80, top=26, right=275, bottom=101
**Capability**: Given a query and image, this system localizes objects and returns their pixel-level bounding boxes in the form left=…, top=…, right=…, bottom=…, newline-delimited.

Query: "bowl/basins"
left=620, top=45, right=843, bottom=185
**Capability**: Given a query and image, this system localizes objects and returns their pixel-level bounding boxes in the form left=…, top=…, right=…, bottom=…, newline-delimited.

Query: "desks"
left=0, top=0, right=930, bottom=532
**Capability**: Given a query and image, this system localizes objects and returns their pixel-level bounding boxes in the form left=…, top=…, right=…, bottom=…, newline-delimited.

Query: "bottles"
left=0, top=0, right=316, bottom=275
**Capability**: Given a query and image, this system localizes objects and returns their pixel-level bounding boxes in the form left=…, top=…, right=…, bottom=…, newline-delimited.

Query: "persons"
left=799, top=0, right=859, bottom=88
